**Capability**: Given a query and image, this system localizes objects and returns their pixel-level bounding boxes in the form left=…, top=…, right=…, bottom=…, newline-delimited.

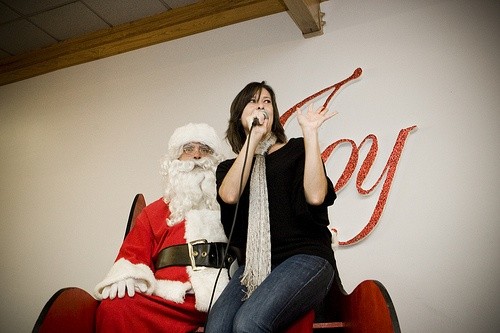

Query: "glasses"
left=183, top=144, right=212, bottom=157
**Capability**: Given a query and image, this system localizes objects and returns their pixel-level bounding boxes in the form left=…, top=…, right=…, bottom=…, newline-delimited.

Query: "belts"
left=155, top=239, right=234, bottom=271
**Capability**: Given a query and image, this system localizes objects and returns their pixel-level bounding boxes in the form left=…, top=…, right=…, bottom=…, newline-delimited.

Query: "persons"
left=204, top=81, right=336, bottom=333
left=93, top=122, right=238, bottom=333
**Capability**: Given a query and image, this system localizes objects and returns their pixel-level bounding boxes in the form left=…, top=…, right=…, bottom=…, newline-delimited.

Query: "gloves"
left=102, top=278, right=148, bottom=300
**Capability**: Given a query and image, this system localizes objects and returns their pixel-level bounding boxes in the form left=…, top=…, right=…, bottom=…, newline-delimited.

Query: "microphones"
left=252, top=110, right=268, bottom=126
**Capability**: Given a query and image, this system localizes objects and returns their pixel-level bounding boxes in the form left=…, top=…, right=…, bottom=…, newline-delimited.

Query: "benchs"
left=31, top=194, right=401, bottom=333
